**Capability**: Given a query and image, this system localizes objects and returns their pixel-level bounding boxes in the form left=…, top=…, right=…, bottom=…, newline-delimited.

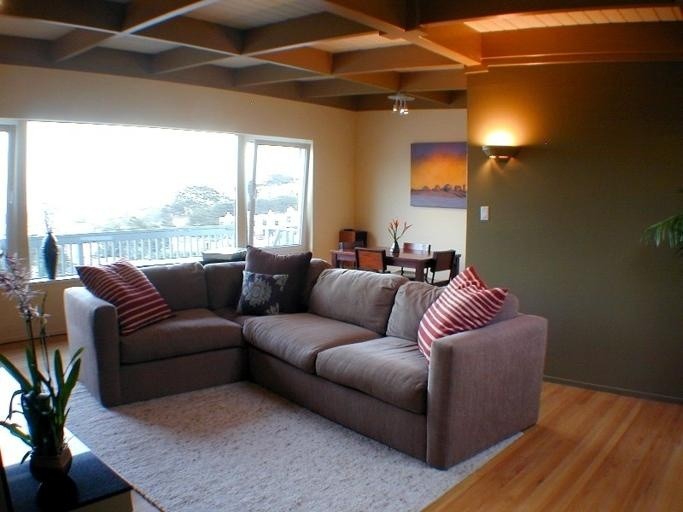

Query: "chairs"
left=354, top=248, right=390, bottom=275
left=338, top=239, right=367, bottom=270
left=390, top=242, right=432, bottom=279
left=427, top=248, right=458, bottom=286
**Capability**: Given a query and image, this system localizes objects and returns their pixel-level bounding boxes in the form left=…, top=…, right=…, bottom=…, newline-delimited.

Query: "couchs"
left=60, top=257, right=548, bottom=473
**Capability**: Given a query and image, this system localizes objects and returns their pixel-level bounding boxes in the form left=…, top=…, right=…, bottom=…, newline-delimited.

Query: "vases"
left=40, top=230, right=60, bottom=280
left=27, top=443, right=72, bottom=485
left=389, top=239, right=400, bottom=252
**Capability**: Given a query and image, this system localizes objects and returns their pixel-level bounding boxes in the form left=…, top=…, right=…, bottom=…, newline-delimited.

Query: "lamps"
left=386, top=94, right=415, bottom=117
left=482, top=144, right=517, bottom=160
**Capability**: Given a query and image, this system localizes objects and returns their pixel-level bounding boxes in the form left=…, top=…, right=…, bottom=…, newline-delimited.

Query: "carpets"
left=32, top=375, right=524, bottom=511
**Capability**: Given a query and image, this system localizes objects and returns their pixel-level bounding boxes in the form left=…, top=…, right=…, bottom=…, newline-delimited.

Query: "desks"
left=329, top=248, right=462, bottom=286
left=0, top=452, right=136, bottom=511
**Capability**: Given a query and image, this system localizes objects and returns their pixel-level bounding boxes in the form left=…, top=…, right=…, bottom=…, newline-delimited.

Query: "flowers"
left=386, top=218, right=413, bottom=242
left=0, top=210, right=84, bottom=467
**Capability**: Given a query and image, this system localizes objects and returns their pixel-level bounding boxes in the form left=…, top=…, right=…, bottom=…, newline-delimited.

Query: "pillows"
left=417, top=263, right=513, bottom=369
left=74, top=257, right=175, bottom=331
left=236, top=269, right=289, bottom=315
left=243, top=242, right=316, bottom=316
left=203, top=250, right=257, bottom=260
